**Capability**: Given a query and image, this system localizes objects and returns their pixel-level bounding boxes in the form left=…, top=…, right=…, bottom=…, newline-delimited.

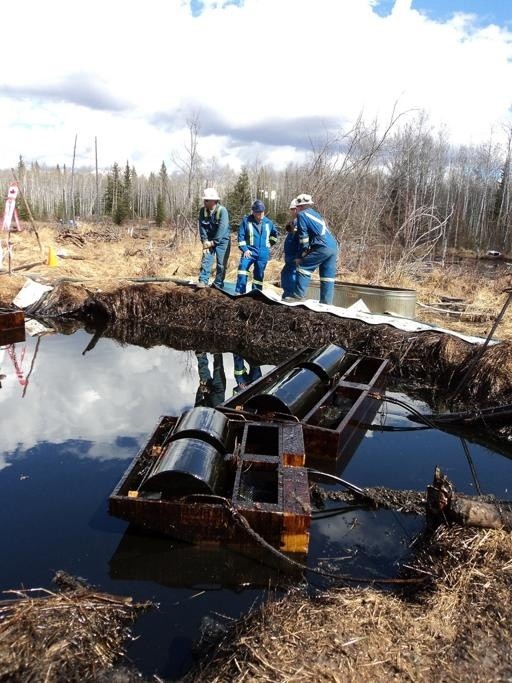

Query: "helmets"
left=252, top=200, right=265, bottom=212
left=295, top=193, right=314, bottom=207
left=288, top=198, right=298, bottom=209
left=201, top=187, right=221, bottom=200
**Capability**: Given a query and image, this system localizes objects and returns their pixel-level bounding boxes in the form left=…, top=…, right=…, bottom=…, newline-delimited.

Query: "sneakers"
left=196, top=281, right=207, bottom=287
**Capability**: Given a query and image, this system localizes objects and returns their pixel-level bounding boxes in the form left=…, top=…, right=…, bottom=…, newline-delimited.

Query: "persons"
left=193, top=350, right=227, bottom=408
left=231, top=353, right=262, bottom=397
left=283, top=193, right=338, bottom=305
left=196, top=187, right=232, bottom=288
left=232, top=200, right=278, bottom=294
left=280, top=198, right=305, bottom=299
